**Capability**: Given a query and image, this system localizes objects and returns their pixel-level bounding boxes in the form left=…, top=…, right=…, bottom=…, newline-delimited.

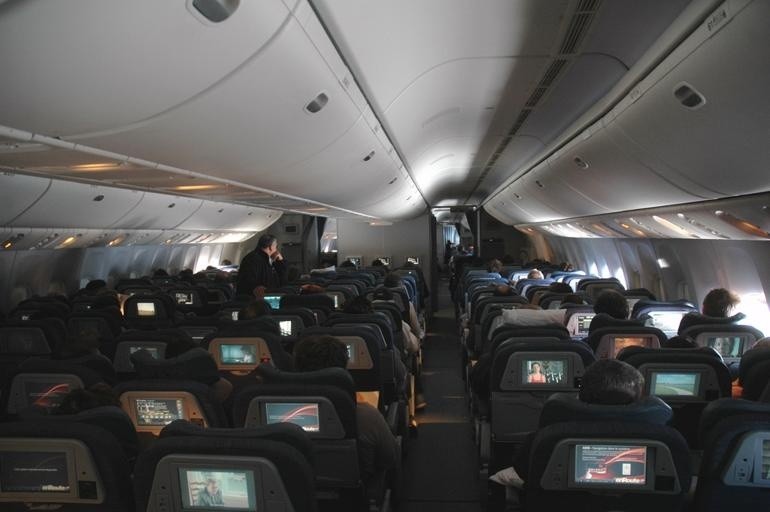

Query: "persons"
left=445, top=241, right=770, bottom=482
left=2, top=259, right=429, bottom=508
left=237, top=235, right=289, bottom=287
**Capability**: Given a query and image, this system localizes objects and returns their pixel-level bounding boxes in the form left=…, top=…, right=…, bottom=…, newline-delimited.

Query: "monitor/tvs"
left=472, top=276, right=748, bottom=385
left=647, top=370, right=702, bottom=400
left=379, top=256, right=391, bottom=267
left=568, top=446, right=656, bottom=491
left=179, top=465, right=258, bottom=512
left=407, top=257, right=420, bottom=266
left=286, top=226, right=297, bottom=234
left=0, top=446, right=78, bottom=497
left=348, top=258, right=361, bottom=268
